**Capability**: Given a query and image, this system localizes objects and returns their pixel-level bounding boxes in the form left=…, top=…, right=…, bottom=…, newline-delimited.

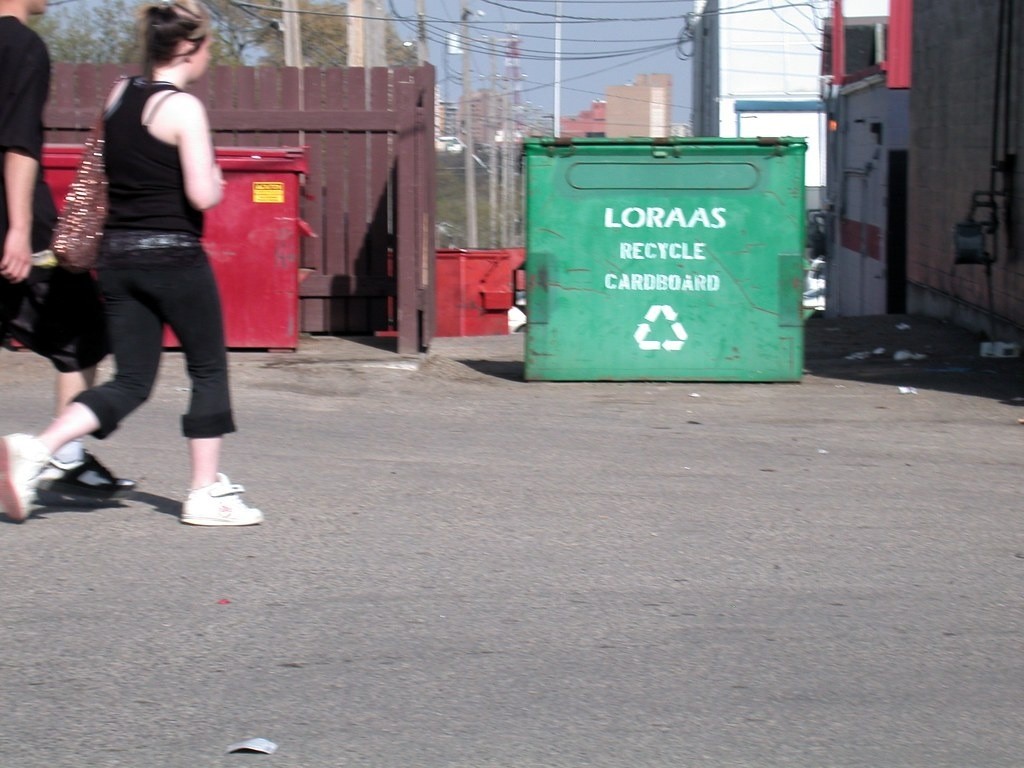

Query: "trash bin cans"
left=41, top=142, right=312, bottom=352
left=434, top=246, right=525, bottom=338
left=515, top=134, right=807, bottom=383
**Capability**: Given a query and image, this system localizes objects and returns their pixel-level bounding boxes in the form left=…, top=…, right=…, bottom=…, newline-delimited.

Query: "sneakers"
left=1, top=432, right=52, bottom=521
left=180, top=470, right=263, bottom=525
left=37, top=453, right=135, bottom=496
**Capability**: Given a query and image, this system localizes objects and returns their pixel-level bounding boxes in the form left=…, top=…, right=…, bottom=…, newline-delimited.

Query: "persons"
left=0, top=0, right=119, bottom=497
left=0, top=0, right=263, bottom=526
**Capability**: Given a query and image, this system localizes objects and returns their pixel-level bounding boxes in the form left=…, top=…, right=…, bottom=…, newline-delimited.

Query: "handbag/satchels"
left=49, top=72, right=126, bottom=273
